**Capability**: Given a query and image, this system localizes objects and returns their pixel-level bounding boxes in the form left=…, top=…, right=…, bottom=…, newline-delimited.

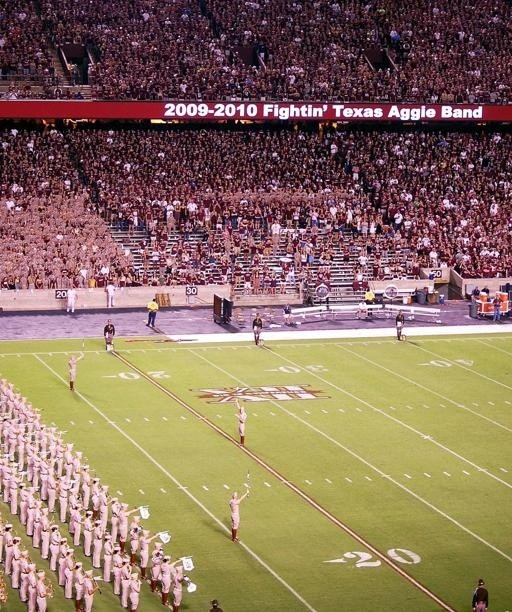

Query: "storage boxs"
left=474, top=291, right=508, bottom=302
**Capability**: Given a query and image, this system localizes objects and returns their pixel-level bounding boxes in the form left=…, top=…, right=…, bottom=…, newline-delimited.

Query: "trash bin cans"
left=469, top=305, right=477, bottom=317
left=417, top=289, right=426, bottom=305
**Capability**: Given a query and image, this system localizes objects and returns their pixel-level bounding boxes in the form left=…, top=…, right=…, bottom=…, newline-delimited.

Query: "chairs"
left=465, top=284, right=475, bottom=300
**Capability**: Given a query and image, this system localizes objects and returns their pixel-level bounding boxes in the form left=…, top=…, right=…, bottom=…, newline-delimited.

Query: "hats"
left=0, top=378, right=189, bottom=579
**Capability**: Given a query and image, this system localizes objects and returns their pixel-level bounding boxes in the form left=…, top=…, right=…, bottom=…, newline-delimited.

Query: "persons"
left=145, top=298, right=159, bottom=327
left=66, top=355, right=82, bottom=391
left=209, top=599, right=223, bottom=612
left=251, top=313, right=263, bottom=345
left=233, top=398, right=247, bottom=446
left=2, top=1, right=512, bottom=324
left=104, top=318, right=115, bottom=350
left=1, top=375, right=188, bottom=612
left=470, top=578, right=490, bottom=612
left=395, top=309, right=405, bottom=342
left=229, top=489, right=249, bottom=543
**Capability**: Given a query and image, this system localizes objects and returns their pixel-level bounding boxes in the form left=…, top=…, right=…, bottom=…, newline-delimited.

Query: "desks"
left=476, top=300, right=509, bottom=312
